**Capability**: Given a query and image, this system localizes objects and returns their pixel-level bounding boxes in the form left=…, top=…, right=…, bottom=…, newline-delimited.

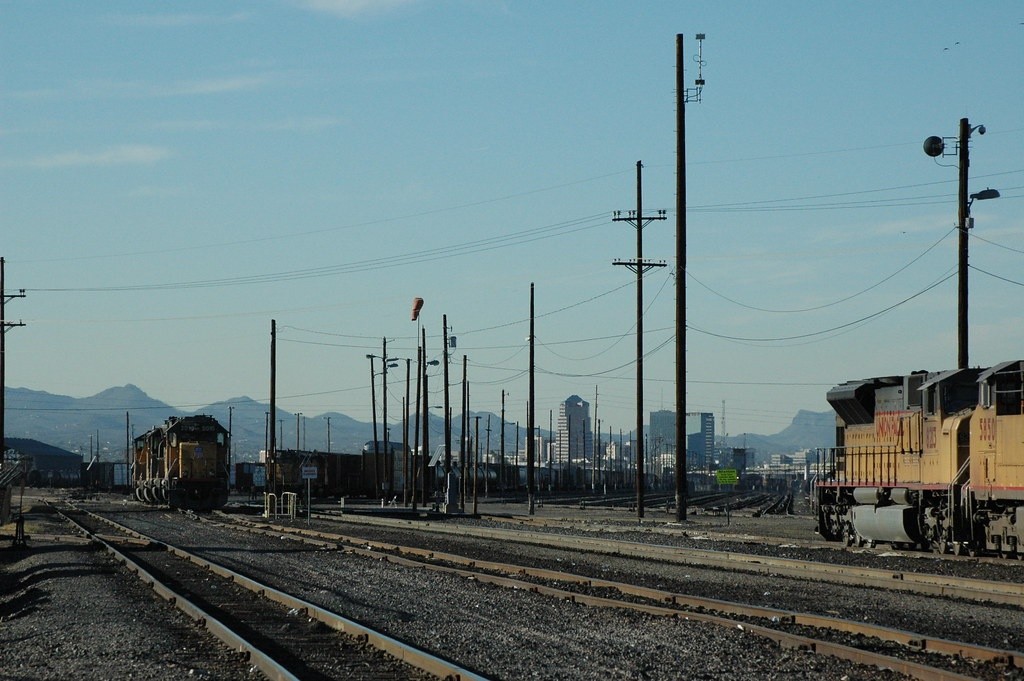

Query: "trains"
left=804, top=357, right=1024, bottom=560
left=131, top=414, right=232, bottom=512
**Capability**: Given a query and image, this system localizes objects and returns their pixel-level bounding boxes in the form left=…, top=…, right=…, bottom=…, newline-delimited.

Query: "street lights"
left=278, top=419, right=286, bottom=450
left=294, top=413, right=303, bottom=450
left=226, top=406, right=235, bottom=468
left=500, top=422, right=516, bottom=489
left=956, top=190, right=1004, bottom=371
left=321, top=416, right=334, bottom=453
left=365, top=352, right=481, bottom=501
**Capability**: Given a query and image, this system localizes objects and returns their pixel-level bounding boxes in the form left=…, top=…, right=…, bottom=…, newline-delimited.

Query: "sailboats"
left=264, top=411, right=271, bottom=478
